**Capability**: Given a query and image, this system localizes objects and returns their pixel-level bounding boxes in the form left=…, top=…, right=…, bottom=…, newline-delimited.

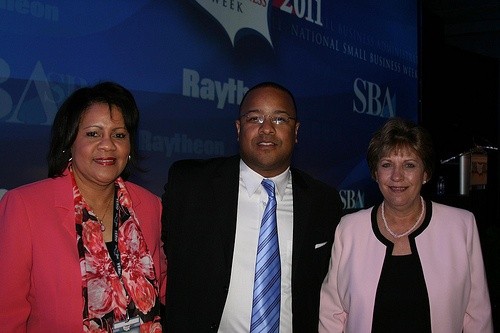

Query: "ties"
left=250, top=178, right=281, bottom=333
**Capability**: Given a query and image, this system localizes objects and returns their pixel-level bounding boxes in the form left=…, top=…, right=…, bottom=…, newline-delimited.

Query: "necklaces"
left=379, top=196, right=423, bottom=238
left=77, top=179, right=118, bottom=231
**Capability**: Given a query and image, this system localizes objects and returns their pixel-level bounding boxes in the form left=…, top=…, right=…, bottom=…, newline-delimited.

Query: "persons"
left=317, top=119, right=493, bottom=333
left=0, top=83, right=167, bottom=333
left=160, top=82, right=343, bottom=333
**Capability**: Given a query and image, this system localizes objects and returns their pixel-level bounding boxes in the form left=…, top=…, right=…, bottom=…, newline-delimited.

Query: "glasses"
left=239, top=113, right=297, bottom=126
